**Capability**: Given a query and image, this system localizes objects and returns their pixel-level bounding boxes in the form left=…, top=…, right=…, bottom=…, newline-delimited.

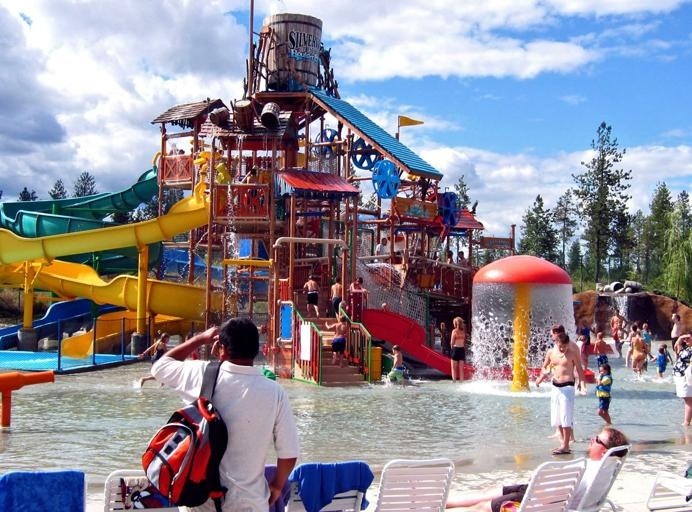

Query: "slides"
left=0, top=167, right=166, bottom=274
left=365, top=306, right=593, bottom=382
left=0, top=167, right=236, bottom=359
left=1, top=296, right=123, bottom=352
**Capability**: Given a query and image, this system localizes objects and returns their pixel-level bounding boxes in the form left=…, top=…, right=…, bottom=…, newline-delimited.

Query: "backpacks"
left=140, top=360, right=230, bottom=512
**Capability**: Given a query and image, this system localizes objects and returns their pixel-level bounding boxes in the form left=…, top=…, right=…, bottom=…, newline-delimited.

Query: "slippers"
left=553, top=449, right=571, bottom=455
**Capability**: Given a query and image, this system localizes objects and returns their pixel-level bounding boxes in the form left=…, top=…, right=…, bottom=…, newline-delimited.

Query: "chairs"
left=646, top=455, right=692, bottom=512
left=375, top=457, right=459, bottom=512
left=290, top=476, right=365, bottom=512
left=516, top=456, right=588, bottom=512
left=103, top=468, right=157, bottom=512
left=571, top=443, right=636, bottom=512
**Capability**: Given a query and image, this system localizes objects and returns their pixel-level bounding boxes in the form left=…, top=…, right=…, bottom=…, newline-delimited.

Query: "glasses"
left=595, top=435, right=610, bottom=450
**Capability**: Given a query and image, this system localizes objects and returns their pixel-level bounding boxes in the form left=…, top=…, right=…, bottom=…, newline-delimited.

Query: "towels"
left=263, top=464, right=291, bottom=512
left=291, top=462, right=371, bottom=512
left=1, top=468, right=86, bottom=512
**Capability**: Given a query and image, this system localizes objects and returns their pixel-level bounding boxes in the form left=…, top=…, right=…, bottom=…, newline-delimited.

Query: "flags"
left=398, top=116, right=424, bottom=126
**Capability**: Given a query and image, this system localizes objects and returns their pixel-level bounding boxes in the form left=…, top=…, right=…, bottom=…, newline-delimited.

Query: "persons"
left=231, top=156, right=281, bottom=212
left=139, top=332, right=170, bottom=388
left=166, top=142, right=224, bottom=159
left=446, top=428, right=629, bottom=512
left=535, top=297, right=692, bottom=455
left=151, top=318, right=298, bottom=512
left=302, top=237, right=473, bottom=384
